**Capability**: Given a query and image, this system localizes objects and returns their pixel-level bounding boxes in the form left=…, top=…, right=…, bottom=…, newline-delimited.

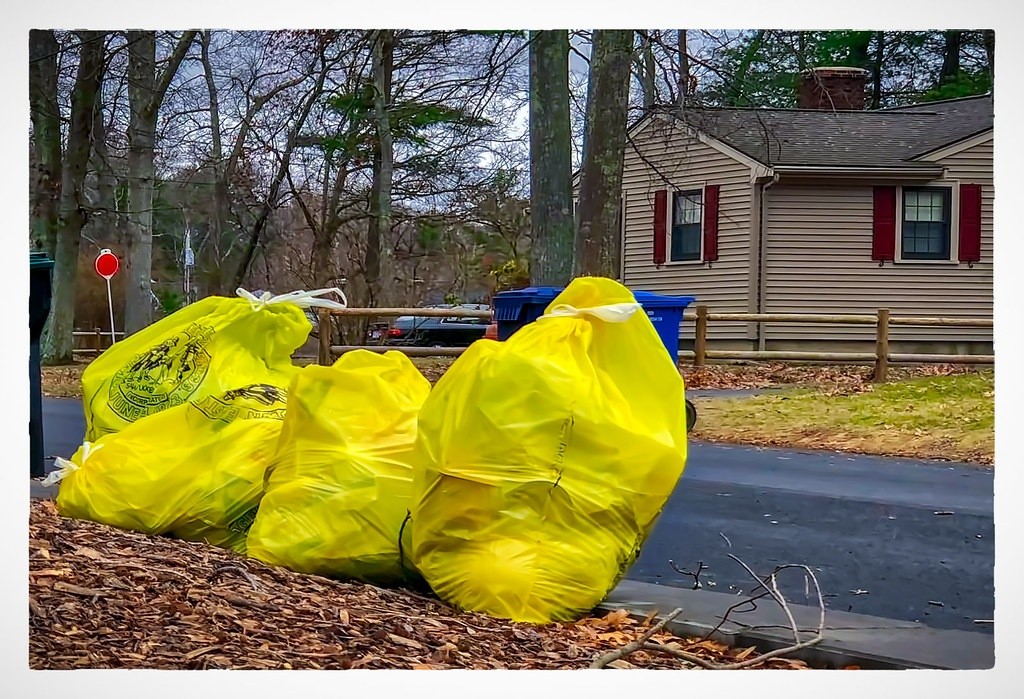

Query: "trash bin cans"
left=29, top=253, right=57, bottom=475
left=630, top=290, right=696, bottom=436
left=491, top=288, right=566, bottom=341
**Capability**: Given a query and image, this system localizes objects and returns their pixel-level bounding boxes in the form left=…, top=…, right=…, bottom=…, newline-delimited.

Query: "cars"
left=387, top=304, right=494, bottom=349
